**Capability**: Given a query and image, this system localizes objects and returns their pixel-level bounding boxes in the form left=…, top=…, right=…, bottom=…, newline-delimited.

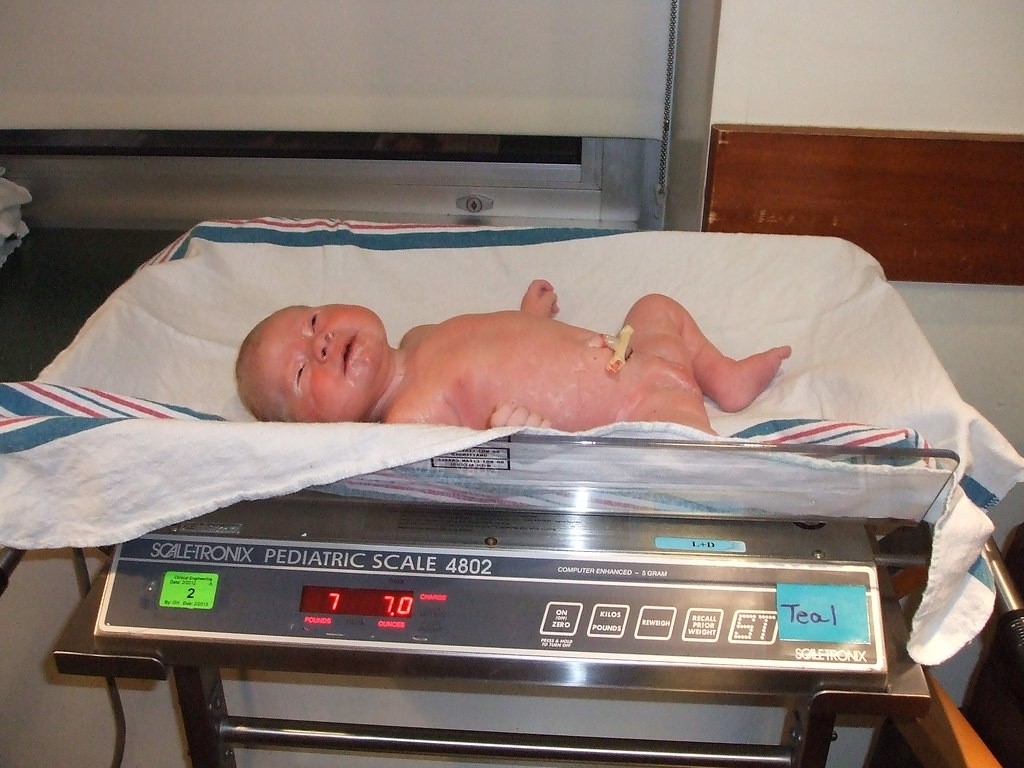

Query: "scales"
left=95, top=219, right=961, bottom=691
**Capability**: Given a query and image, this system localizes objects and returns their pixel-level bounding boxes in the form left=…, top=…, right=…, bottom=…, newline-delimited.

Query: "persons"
left=235, top=278, right=793, bottom=444
